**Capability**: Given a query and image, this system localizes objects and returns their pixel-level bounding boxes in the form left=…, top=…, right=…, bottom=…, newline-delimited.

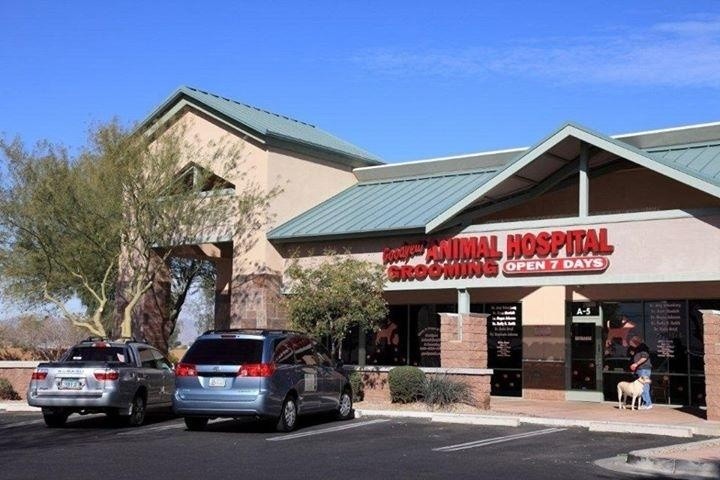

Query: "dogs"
left=616, top=376, right=652, bottom=411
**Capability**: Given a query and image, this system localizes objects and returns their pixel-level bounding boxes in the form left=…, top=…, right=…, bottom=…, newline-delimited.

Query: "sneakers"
left=638, top=405, right=654, bottom=412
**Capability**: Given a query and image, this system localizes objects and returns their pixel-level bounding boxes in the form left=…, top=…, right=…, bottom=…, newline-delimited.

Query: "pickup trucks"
left=26, top=335, right=177, bottom=427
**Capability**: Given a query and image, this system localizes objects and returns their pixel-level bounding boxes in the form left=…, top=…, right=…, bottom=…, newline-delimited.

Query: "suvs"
left=173, top=327, right=353, bottom=433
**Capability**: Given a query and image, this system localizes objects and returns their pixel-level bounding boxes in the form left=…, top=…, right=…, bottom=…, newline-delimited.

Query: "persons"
left=630, top=338, right=652, bottom=409
